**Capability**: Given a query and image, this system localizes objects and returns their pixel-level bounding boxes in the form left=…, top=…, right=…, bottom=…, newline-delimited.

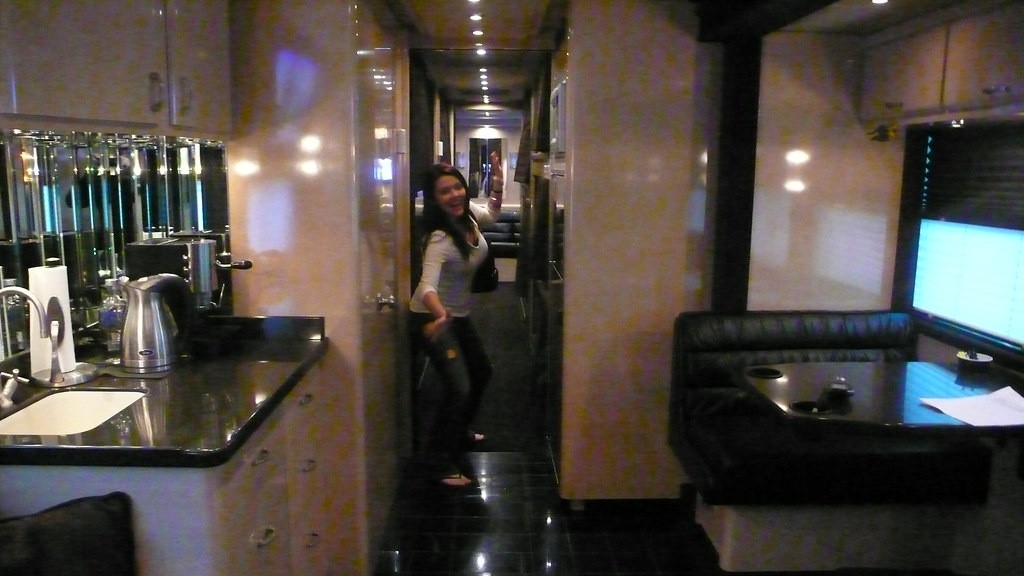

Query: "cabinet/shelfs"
left=0, top=362, right=329, bottom=576
left=0, top=0, right=233, bottom=140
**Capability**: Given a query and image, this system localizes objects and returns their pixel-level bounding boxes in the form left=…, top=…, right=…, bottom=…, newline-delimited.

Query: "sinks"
left=0, top=391, right=143, bottom=435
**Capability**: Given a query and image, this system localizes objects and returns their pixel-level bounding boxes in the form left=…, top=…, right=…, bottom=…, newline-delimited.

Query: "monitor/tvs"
left=911, top=217, right=1024, bottom=356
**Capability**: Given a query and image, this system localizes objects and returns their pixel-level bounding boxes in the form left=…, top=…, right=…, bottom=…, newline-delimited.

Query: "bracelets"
left=492, top=190, right=502, bottom=193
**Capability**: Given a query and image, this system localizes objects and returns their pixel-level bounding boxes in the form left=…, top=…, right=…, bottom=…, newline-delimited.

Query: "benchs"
left=666, top=311, right=996, bottom=572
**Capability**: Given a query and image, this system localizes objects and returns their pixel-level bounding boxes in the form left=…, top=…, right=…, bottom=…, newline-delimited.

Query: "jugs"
left=120, top=273, right=187, bottom=374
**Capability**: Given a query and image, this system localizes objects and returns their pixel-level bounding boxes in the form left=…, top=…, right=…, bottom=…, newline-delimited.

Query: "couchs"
left=481, top=212, right=521, bottom=257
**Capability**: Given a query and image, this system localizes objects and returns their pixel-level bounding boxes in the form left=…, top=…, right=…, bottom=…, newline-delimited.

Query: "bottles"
left=0, top=279, right=27, bottom=356
left=98, top=279, right=128, bottom=364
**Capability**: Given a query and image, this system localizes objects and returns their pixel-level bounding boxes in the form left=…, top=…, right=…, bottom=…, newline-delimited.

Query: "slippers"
left=432, top=472, right=474, bottom=492
left=460, top=430, right=488, bottom=444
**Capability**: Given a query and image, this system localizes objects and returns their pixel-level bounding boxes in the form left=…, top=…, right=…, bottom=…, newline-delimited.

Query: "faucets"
left=1, top=286, right=49, bottom=339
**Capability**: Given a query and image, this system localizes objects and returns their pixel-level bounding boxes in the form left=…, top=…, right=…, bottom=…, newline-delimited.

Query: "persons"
left=409, top=151, right=504, bottom=493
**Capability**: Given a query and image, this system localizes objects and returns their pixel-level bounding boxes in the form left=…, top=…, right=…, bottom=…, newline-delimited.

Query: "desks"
left=743, top=361, right=1024, bottom=438
